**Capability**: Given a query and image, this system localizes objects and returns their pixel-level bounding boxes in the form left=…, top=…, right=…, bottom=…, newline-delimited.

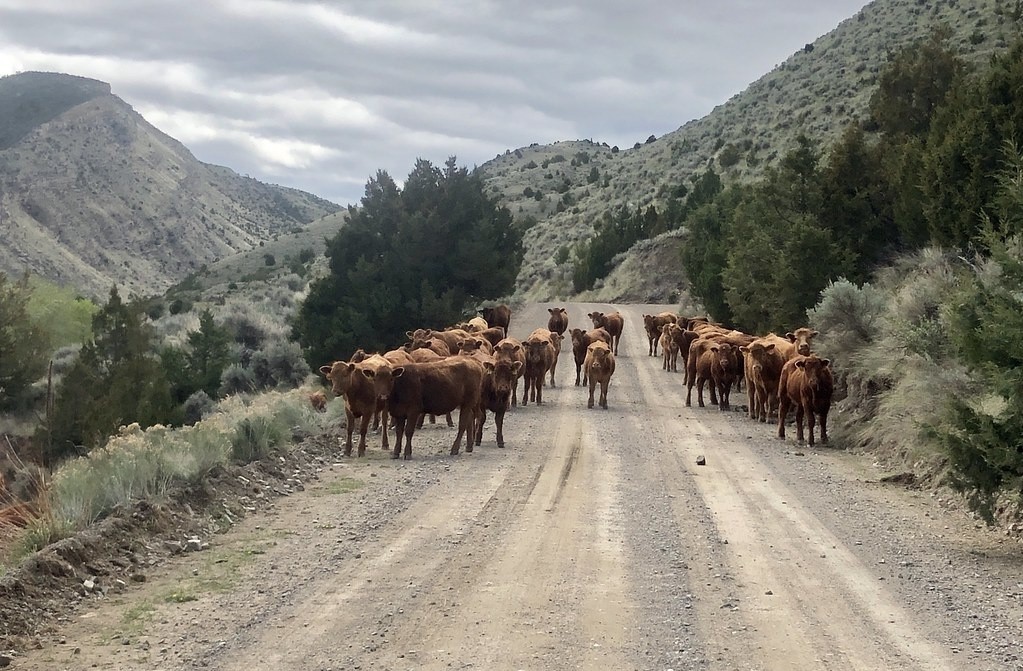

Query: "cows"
left=320, top=304, right=836, bottom=460
